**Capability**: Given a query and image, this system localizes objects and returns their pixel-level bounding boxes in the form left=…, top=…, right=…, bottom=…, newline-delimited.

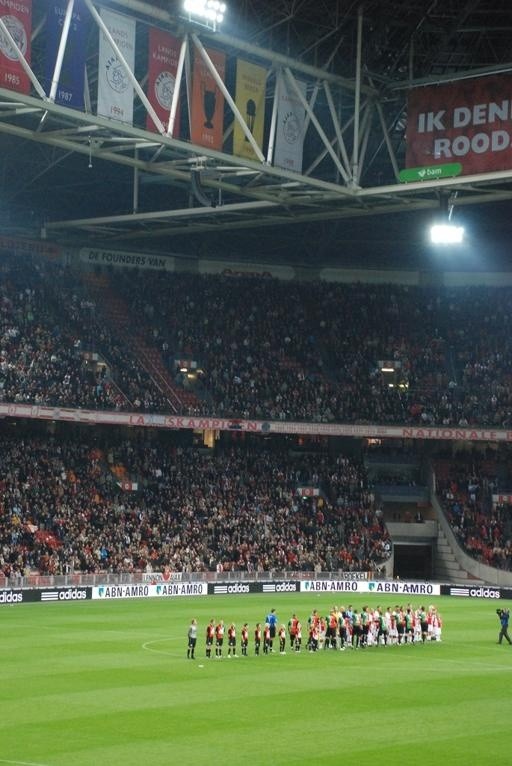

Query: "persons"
left=278, top=623, right=287, bottom=655
left=0, top=246, right=512, bottom=585
left=266, top=608, right=278, bottom=652
left=227, top=623, right=239, bottom=657
left=294, top=621, right=303, bottom=652
left=205, top=619, right=215, bottom=658
left=288, top=613, right=298, bottom=650
left=187, top=618, right=197, bottom=660
left=241, top=623, right=250, bottom=656
left=215, top=619, right=226, bottom=658
left=306, top=602, right=443, bottom=652
left=254, top=623, right=261, bottom=656
left=495, top=606, right=512, bottom=645
left=264, top=622, right=271, bottom=655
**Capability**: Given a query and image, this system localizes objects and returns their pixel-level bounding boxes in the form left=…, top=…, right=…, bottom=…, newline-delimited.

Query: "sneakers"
left=509, top=642, right=512, bottom=645
left=496, top=641, right=502, bottom=645
left=354, top=642, right=425, bottom=649
left=186, top=653, right=270, bottom=660
left=269, top=646, right=346, bottom=654
